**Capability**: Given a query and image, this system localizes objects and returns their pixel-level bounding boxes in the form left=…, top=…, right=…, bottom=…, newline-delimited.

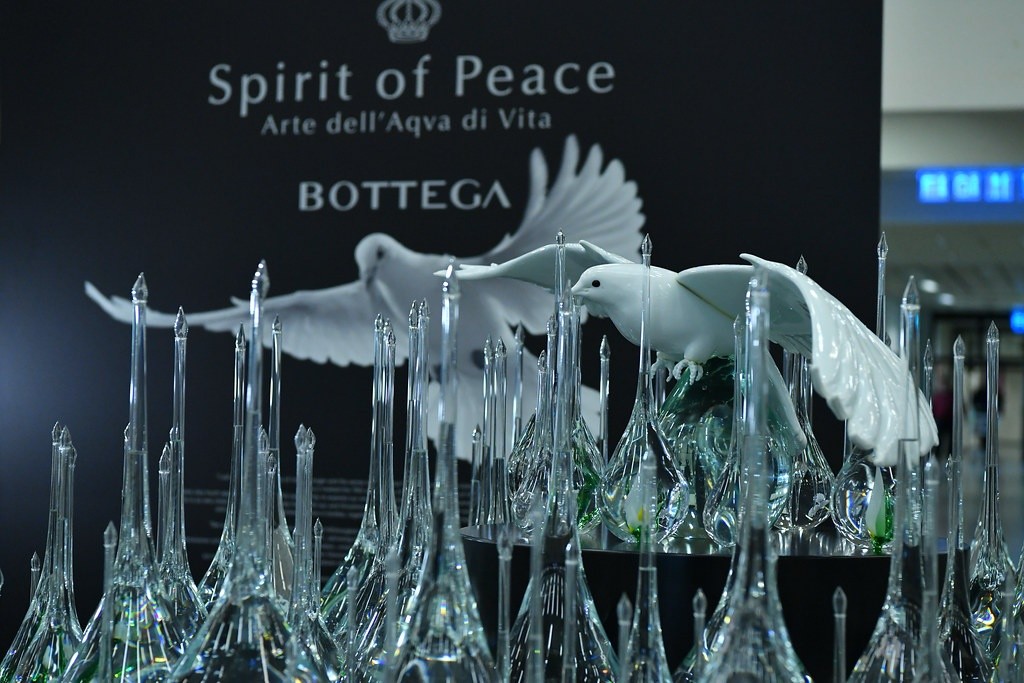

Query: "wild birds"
left=434, top=239, right=940, bottom=464
left=78, top=129, right=645, bottom=468
